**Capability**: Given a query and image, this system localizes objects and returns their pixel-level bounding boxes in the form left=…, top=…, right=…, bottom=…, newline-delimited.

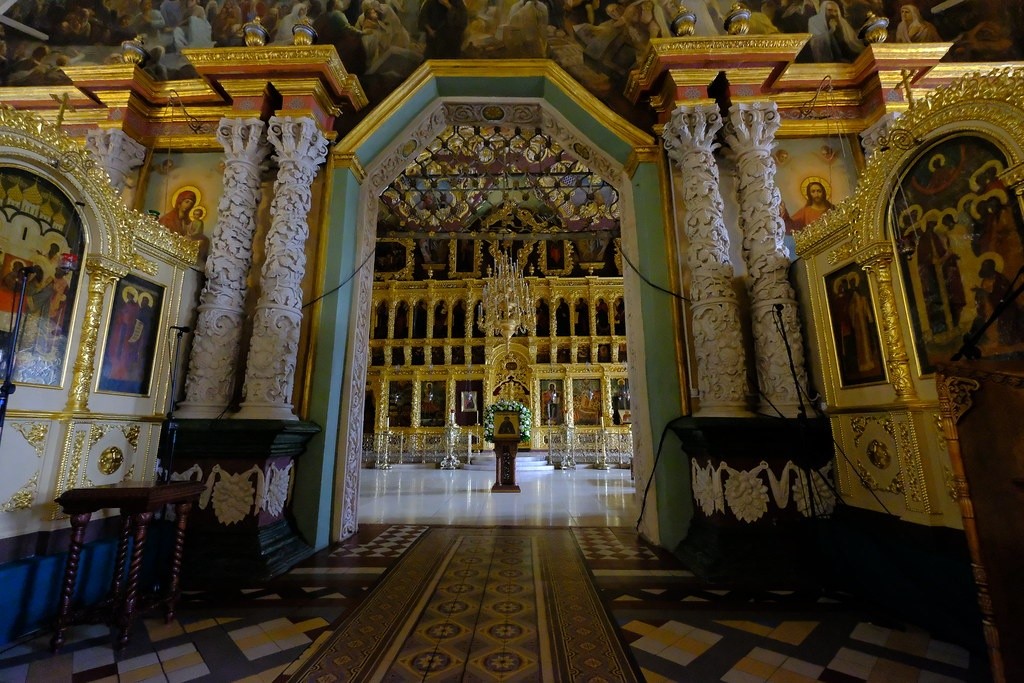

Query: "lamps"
left=477, top=233, right=535, bottom=353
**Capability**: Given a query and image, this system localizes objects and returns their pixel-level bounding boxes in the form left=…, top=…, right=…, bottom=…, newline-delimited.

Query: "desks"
left=48, top=480, right=208, bottom=660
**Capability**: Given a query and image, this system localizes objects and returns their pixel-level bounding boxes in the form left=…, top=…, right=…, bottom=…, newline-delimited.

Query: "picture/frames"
left=461, top=390, right=478, bottom=412
left=820, top=258, right=892, bottom=390
left=93, top=271, right=169, bottom=398
left=618, top=409, right=631, bottom=423
left=493, top=410, right=520, bottom=438
